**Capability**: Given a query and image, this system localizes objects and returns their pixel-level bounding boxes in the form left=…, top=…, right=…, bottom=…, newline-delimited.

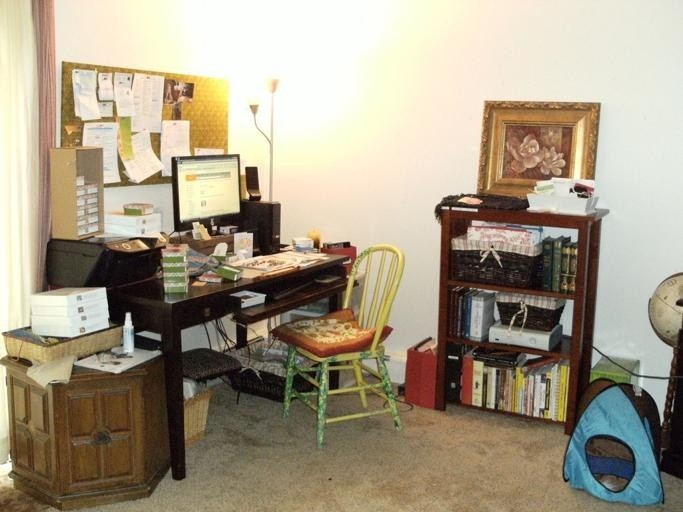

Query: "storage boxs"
left=487, top=317, right=562, bottom=353
left=282, top=272, right=367, bottom=392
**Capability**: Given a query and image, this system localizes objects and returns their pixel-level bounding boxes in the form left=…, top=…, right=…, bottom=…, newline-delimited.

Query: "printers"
left=44, top=233, right=168, bottom=288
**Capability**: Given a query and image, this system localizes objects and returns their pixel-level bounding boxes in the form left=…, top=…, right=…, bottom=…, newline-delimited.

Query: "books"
left=444, top=224, right=579, bottom=423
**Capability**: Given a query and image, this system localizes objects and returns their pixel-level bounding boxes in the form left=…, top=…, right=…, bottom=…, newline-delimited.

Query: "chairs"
left=268, top=243, right=404, bottom=451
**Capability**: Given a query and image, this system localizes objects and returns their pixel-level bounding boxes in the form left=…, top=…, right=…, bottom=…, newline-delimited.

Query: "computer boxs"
left=223, top=201, right=281, bottom=255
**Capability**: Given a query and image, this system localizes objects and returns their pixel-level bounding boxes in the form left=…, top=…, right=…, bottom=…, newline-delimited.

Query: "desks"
left=106, top=250, right=357, bottom=481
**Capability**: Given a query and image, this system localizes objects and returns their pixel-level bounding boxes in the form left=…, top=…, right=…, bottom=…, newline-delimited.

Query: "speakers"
left=245, top=166, right=261, bottom=201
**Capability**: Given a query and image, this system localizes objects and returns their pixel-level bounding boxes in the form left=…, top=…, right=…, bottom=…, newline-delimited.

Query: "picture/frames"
left=475, top=99, right=601, bottom=201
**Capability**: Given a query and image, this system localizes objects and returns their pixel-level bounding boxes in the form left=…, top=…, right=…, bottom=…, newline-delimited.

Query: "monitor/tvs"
left=171, top=154, right=241, bottom=232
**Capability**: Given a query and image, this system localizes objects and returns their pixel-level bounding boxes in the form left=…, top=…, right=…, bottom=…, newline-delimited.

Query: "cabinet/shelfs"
left=0, top=348, right=172, bottom=512
left=431, top=205, right=606, bottom=437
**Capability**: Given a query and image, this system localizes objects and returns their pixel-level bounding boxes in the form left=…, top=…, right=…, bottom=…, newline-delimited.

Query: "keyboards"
left=265, top=275, right=314, bottom=300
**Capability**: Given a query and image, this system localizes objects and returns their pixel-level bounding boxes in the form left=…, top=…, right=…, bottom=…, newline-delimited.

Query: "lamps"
left=249, top=71, right=278, bottom=199
